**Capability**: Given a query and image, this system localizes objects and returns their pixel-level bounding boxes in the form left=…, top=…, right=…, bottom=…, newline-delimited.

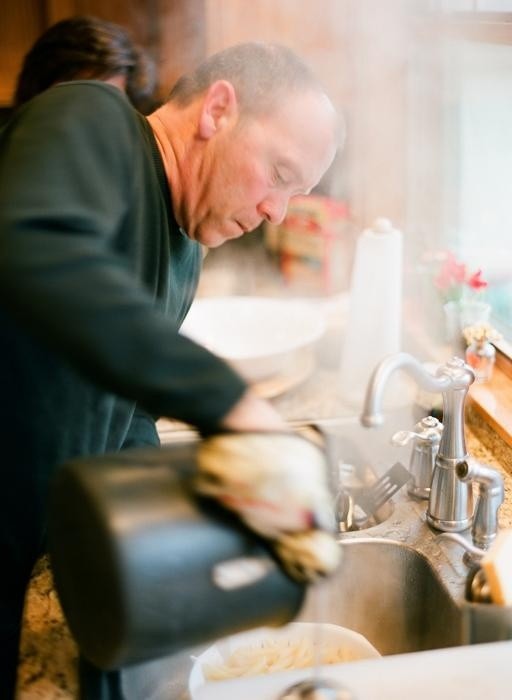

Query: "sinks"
left=298, top=540, right=512, bottom=662
left=304, top=416, right=412, bottom=538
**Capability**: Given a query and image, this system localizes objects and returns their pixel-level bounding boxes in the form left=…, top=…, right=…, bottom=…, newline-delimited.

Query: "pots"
left=45, top=440, right=306, bottom=671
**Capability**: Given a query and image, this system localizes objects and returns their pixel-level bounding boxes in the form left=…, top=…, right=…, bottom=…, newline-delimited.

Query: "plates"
left=186, top=622, right=380, bottom=697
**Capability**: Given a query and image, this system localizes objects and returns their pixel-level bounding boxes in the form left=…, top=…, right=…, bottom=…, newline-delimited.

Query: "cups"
left=444, top=298, right=489, bottom=353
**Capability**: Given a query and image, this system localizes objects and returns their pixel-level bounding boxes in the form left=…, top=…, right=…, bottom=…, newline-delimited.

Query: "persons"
left=1, top=42, right=338, bottom=700
left=15, top=16, right=156, bottom=108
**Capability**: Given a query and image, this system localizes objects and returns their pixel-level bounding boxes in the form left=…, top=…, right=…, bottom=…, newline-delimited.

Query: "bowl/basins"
left=211, top=325, right=321, bottom=384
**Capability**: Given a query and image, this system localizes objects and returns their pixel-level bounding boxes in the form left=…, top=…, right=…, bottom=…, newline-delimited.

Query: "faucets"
left=362, top=351, right=477, bottom=533
left=453, top=460, right=503, bottom=546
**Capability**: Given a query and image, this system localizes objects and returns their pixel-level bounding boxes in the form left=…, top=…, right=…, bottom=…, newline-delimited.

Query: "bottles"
left=467, top=335, right=496, bottom=379
